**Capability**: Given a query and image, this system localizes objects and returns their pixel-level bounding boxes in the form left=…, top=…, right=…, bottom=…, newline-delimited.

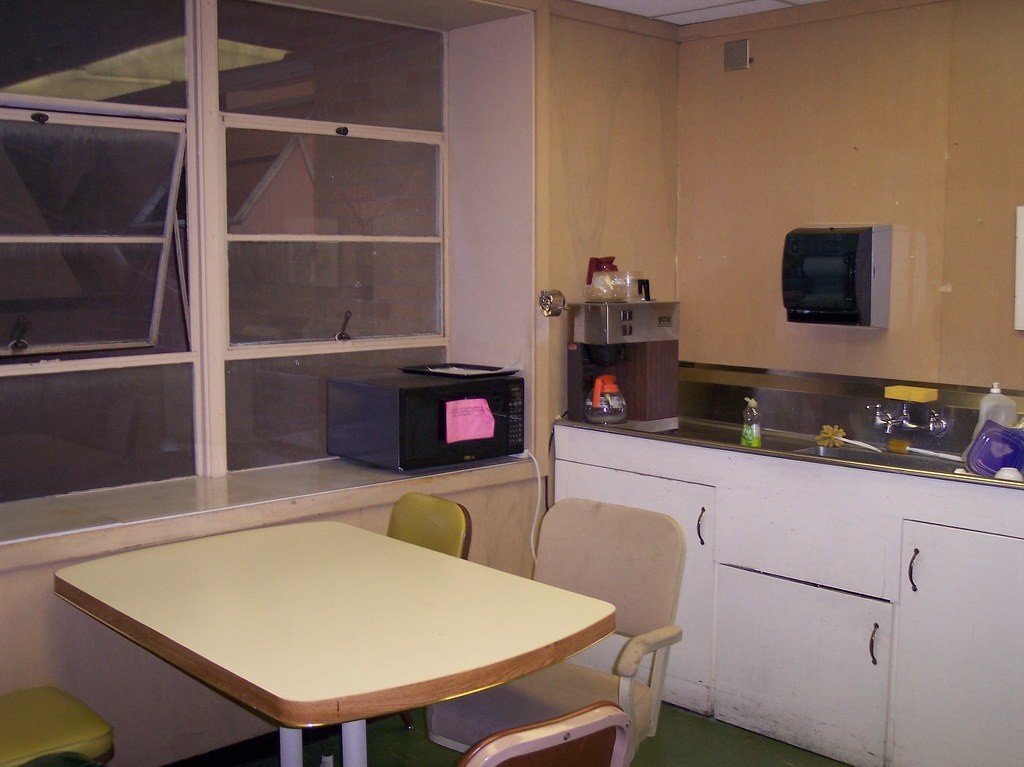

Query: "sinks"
left=795, top=445, right=965, bottom=472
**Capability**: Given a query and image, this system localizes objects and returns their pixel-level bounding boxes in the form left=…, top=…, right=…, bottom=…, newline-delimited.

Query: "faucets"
left=861, top=398, right=949, bottom=439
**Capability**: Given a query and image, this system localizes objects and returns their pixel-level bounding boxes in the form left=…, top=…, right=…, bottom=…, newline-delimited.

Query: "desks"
left=54, top=519, right=618, bottom=766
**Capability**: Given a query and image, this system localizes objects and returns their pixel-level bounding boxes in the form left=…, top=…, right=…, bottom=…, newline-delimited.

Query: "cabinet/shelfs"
left=713, top=563, right=893, bottom=767
left=886, top=518, right=1024, bottom=766
left=554, top=458, right=717, bottom=718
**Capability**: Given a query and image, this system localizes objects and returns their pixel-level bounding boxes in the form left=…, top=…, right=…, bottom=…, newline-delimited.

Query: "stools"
left=0, top=687, right=114, bottom=767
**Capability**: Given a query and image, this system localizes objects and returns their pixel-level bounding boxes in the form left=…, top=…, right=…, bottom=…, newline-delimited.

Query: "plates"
left=394, top=359, right=519, bottom=376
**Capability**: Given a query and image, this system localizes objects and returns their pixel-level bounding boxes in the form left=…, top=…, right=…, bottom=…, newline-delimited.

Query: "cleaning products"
left=740, top=396, right=762, bottom=449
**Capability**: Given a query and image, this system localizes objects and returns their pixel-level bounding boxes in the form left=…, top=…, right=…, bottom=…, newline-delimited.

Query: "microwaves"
left=323, top=378, right=524, bottom=475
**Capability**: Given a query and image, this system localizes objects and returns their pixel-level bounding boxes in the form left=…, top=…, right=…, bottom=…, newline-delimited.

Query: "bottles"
left=972, top=382, right=1016, bottom=442
left=741, top=399, right=761, bottom=448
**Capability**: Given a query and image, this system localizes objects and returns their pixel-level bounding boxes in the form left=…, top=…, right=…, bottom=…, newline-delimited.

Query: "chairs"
left=425, top=498, right=686, bottom=753
left=303, top=493, right=474, bottom=767
left=454, top=701, right=634, bottom=767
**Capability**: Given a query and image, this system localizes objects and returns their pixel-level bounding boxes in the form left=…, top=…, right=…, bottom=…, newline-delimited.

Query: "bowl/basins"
left=992, top=467, right=1024, bottom=480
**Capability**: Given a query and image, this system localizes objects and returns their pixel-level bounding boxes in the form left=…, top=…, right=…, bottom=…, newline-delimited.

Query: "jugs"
left=584, top=374, right=626, bottom=425
left=582, top=254, right=625, bottom=302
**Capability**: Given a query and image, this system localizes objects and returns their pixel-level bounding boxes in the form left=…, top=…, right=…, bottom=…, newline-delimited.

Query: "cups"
left=622, top=267, right=652, bottom=302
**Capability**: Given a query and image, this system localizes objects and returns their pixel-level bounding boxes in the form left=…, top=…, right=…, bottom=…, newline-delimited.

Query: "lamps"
left=1, top=32, right=287, bottom=99
